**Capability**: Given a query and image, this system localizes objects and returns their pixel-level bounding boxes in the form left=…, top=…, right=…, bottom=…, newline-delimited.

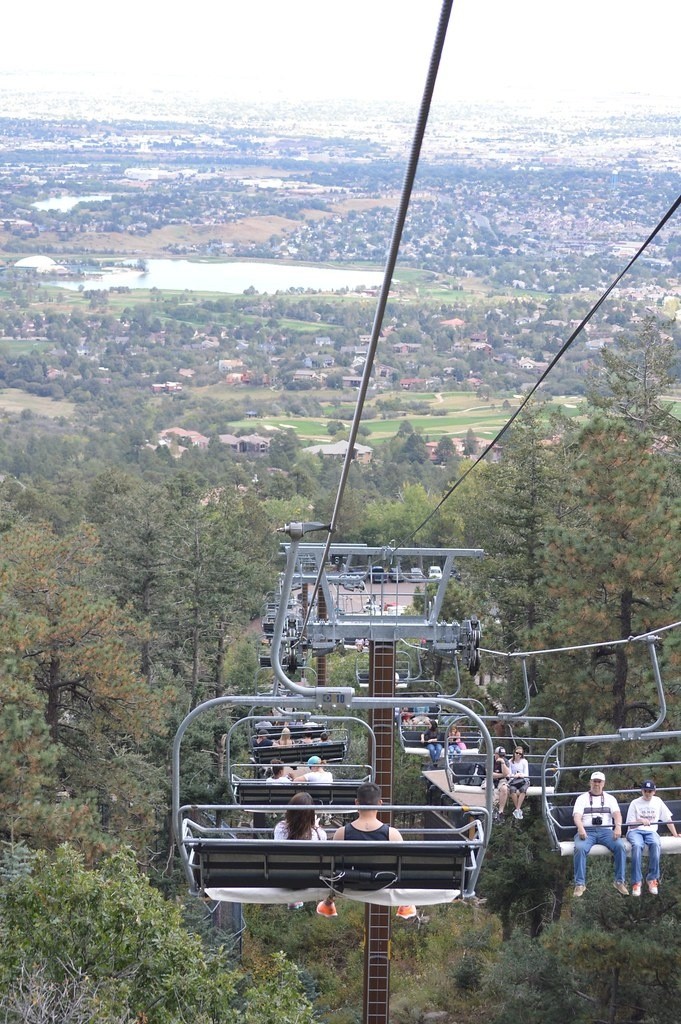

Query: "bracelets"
left=615, top=823, right=621, bottom=826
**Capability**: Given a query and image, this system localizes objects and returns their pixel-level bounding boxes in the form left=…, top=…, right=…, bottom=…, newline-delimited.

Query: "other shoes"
left=495, top=811, right=506, bottom=825
left=450, top=760, right=453, bottom=765
left=458, top=760, right=462, bottom=763
left=433, top=760, right=439, bottom=767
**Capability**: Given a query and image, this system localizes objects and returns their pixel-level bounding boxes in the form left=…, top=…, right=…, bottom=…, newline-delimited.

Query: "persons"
left=626, top=780, right=681, bottom=896
left=294, top=756, right=333, bottom=782
left=252, top=729, right=273, bottom=747
left=273, top=792, right=327, bottom=909
left=266, top=758, right=294, bottom=783
left=485, top=746, right=511, bottom=816
left=317, top=783, right=417, bottom=919
left=355, top=638, right=364, bottom=652
left=447, top=725, right=462, bottom=769
left=509, top=745, right=530, bottom=820
left=317, top=733, right=333, bottom=746
left=334, top=639, right=346, bottom=657
left=572, top=771, right=629, bottom=897
left=273, top=727, right=295, bottom=745
left=421, top=721, right=442, bottom=767
left=400, top=706, right=432, bottom=731
left=299, top=728, right=317, bottom=747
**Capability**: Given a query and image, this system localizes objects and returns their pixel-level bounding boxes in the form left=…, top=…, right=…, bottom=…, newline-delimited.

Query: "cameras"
left=592, top=818, right=602, bottom=825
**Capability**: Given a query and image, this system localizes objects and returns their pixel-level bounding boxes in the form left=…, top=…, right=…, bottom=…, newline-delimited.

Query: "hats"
left=639, top=780, right=655, bottom=790
left=495, top=746, right=506, bottom=756
left=308, top=755, right=321, bottom=766
left=590, top=771, right=606, bottom=781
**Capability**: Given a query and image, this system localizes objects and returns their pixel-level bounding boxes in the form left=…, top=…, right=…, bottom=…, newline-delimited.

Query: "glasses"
left=515, top=752, right=522, bottom=756
left=498, top=755, right=505, bottom=757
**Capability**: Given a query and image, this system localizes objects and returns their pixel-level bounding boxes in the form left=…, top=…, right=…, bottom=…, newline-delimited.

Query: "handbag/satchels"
left=509, top=772, right=526, bottom=789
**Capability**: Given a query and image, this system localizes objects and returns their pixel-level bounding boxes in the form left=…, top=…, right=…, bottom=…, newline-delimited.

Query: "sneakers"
left=632, top=880, right=643, bottom=897
left=396, top=905, right=417, bottom=919
left=613, top=882, right=630, bottom=895
left=288, top=901, right=303, bottom=910
left=647, top=879, right=659, bottom=894
left=316, top=901, right=338, bottom=918
left=573, top=885, right=586, bottom=897
left=512, top=808, right=523, bottom=820
